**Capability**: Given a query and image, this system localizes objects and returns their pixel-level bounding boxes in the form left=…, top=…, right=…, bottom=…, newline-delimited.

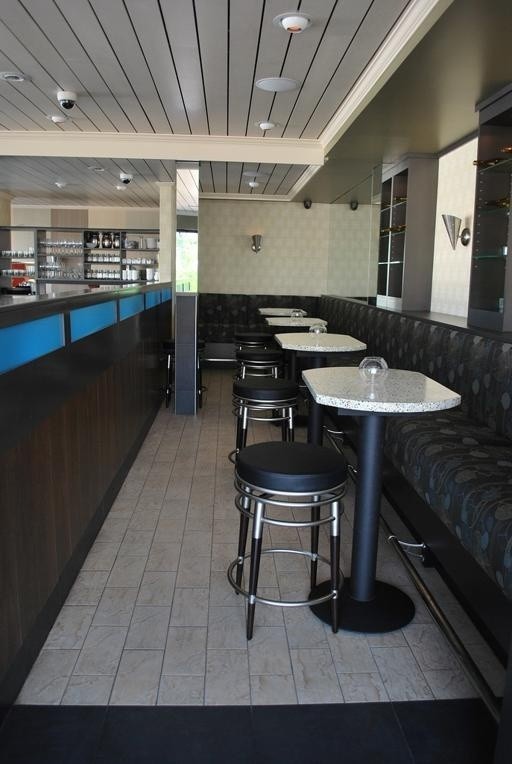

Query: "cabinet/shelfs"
left=0, top=225, right=159, bottom=297
left=467, top=84, right=512, bottom=335
left=376, top=152, right=439, bottom=312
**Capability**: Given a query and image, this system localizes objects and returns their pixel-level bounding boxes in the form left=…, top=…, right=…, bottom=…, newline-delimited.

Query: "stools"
left=230, top=332, right=350, bottom=642
left=161, top=339, right=207, bottom=409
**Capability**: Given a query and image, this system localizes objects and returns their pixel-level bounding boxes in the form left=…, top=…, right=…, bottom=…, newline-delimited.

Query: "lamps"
left=251, top=235, right=263, bottom=253
left=442, top=214, right=471, bottom=250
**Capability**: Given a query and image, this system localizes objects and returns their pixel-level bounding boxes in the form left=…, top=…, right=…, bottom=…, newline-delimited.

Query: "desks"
left=258, top=307, right=463, bottom=633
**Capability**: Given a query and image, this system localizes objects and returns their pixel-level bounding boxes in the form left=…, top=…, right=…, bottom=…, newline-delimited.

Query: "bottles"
left=380, top=195, right=406, bottom=235
left=85, top=253, right=121, bottom=280
left=484, top=198, right=509, bottom=208
left=473, top=158, right=502, bottom=168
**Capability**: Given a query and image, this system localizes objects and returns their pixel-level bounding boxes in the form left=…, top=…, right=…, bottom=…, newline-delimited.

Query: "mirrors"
left=327, top=174, right=382, bottom=306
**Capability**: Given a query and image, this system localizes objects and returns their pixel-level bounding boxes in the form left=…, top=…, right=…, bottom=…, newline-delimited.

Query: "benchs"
left=196, top=293, right=320, bottom=362
left=318, top=294, right=511, bottom=729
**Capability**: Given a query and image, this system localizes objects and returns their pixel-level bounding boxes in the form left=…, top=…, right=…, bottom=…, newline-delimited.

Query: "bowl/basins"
left=122, top=268, right=159, bottom=281
left=123, top=238, right=158, bottom=249
left=86, top=243, right=97, bottom=249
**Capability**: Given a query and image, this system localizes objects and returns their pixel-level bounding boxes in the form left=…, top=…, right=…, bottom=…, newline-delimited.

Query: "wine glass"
left=38, top=239, right=83, bottom=280
